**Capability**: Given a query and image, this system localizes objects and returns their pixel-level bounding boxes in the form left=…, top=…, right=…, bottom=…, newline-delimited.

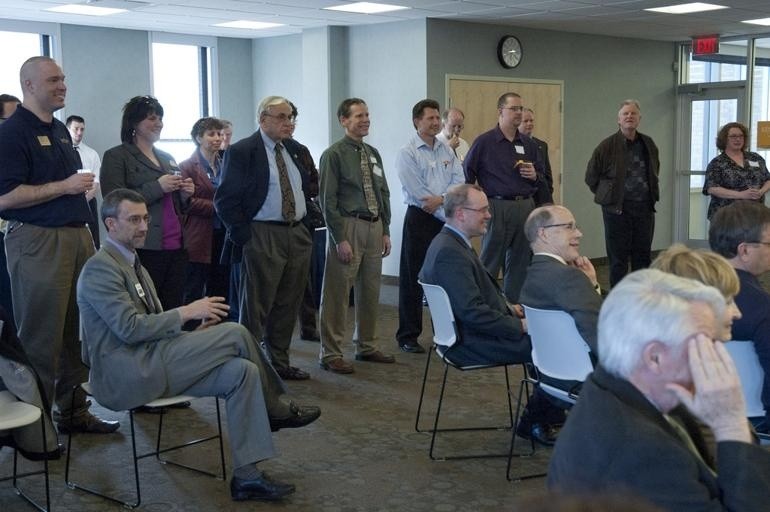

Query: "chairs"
left=505, top=302, right=595, bottom=482
left=719, top=339, right=769, bottom=448
left=0, top=316, right=51, bottom=512
left=64, top=341, right=226, bottom=508
left=415, top=277, right=535, bottom=461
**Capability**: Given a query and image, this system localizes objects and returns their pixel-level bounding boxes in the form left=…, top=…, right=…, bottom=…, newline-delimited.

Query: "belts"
left=494, top=195, right=529, bottom=200
left=266, top=218, right=304, bottom=226
left=356, top=215, right=378, bottom=221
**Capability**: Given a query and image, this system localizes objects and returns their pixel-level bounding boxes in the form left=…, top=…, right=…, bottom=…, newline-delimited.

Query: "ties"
left=134, top=256, right=155, bottom=309
left=276, top=143, right=295, bottom=219
left=360, top=146, right=378, bottom=217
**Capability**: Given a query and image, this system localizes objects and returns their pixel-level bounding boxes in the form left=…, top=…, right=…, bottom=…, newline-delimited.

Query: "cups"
left=77, top=168, right=93, bottom=173
left=518, top=161, right=532, bottom=169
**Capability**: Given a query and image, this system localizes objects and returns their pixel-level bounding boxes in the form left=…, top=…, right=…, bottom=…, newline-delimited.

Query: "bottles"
left=170, top=166, right=183, bottom=192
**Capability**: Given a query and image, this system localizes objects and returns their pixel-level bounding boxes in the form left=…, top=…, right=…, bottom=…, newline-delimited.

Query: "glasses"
left=544, top=224, right=577, bottom=231
left=264, top=113, right=292, bottom=121
left=464, top=206, right=488, bottom=213
left=109, top=214, right=151, bottom=224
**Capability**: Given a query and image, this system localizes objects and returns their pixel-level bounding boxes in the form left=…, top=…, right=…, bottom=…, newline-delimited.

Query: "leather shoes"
left=516, top=419, right=560, bottom=444
left=402, top=342, right=423, bottom=352
left=58, top=412, right=120, bottom=434
left=285, top=366, right=310, bottom=380
left=230, top=472, right=295, bottom=501
left=320, top=359, right=353, bottom=374
left=270, top=400, right=321, bottom=430
left=356, top=351, right=393, bottom=362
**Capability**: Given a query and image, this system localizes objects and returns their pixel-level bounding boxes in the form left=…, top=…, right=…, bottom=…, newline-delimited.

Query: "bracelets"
left=591, top=284, right=599, bottom=289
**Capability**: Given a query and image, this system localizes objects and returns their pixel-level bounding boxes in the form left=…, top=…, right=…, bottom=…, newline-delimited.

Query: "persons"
left=702, top=123, right=769, bottom=220
left=395, top=99, right=465, bottom=352
left=321, top=98, right=395, bottom=372
left=66, top=116, right=101, bottom=252
left=518, top=205, right=604, bottom=395
left=289, top=102, right=321, bottom=343
left=585, top=100, right=660, bottom=290
left=1, top=56, right=120, bottom=460
left=435, top=108, right=469, bottom=164
left=76, top=189, right=320, bottom=502
left=517, top=109, right=554, bottom=208
left=548, top=268, right=770, bottom=510
left=419, top=184, right=567, bottom=447
left=708, top=199, right=769, bottom=413
left=217, top=120, right=233, bottom=162
left=99, top=96, right=191, bottom=414
left=460, top=91, right=545, bottom=305
left=649, top=244, right=770, bottom=472
left=176, top=117, right=229, bottom=332
left=1, top=95, right=21, bottom=334
left=212, top=97, right=311, bottom=380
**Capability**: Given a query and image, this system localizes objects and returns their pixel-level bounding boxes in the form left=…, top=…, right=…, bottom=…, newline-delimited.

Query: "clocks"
left=497, top=35, right=524, bottom=69
